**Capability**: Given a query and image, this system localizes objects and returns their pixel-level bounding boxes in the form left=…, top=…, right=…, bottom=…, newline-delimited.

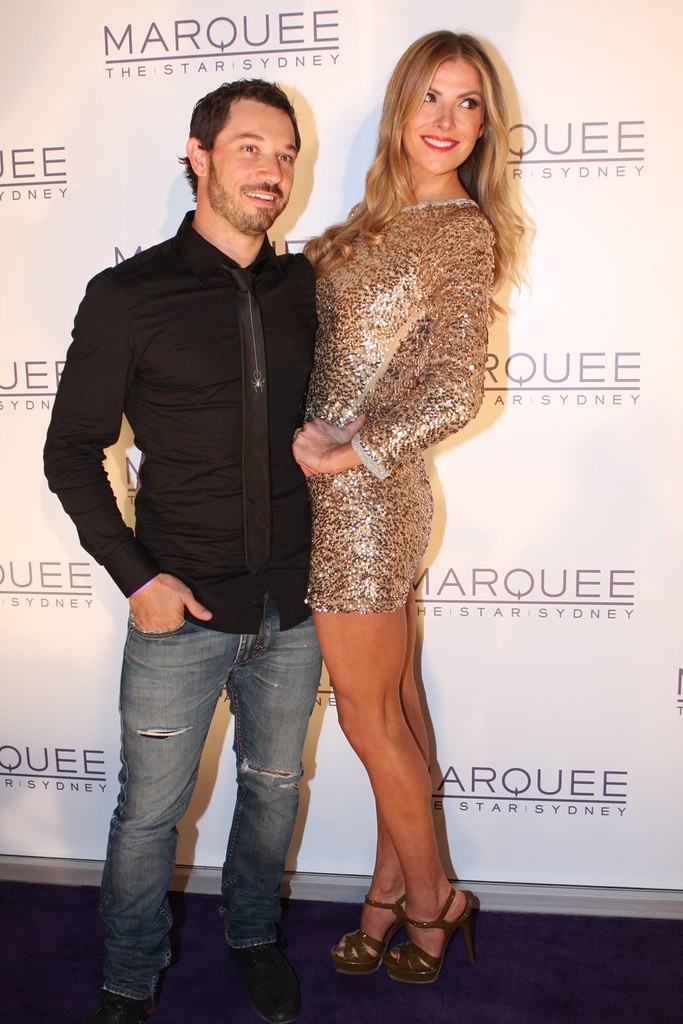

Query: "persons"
left=300, top=31, right=525, bottom=988
left=45, top=80, right=321, bottom=1023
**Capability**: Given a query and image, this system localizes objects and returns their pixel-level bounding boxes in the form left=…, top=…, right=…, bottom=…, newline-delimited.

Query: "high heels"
left=386, top=887, right=475, bottom=984
left=331, top=893, right=406, bottom=974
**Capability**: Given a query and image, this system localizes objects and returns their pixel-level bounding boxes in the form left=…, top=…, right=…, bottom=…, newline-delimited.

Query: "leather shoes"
left=229, top=944, right=301, bottom=1024
left=85, top=987, right=158, bottom=1024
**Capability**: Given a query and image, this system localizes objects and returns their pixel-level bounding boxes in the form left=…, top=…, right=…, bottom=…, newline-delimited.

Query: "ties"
left=220, top=266, right=270, bottom=569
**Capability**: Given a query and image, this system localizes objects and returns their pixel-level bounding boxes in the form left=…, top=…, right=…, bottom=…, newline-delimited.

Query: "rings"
left=292, top=428, right=302, bottom=441
